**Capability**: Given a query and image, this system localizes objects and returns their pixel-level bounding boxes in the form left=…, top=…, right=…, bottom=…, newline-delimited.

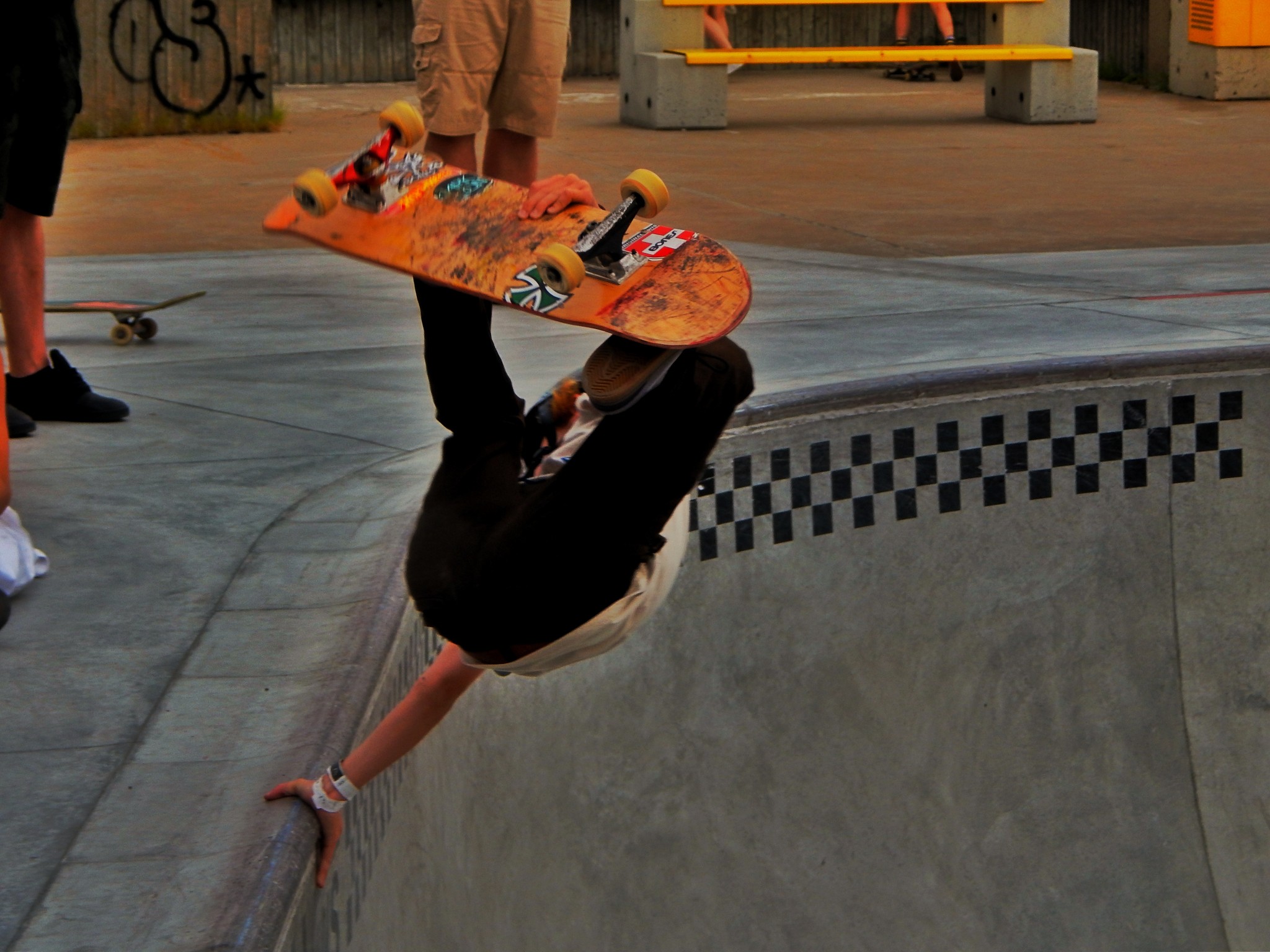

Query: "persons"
left=1, top=0, right=130, bottom=437
left=702, top=2, right=744, bottom=75
left=411, top=0, right=572, bottom=187
left=884, top=0, right=964, bottom=82
left=263, top=172, right=756, bottom=890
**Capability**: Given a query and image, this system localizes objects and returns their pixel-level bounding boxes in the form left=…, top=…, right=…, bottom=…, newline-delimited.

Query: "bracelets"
left=326, top=759, right=359, bottom=801
left=313, top=773, right=347, bottom=813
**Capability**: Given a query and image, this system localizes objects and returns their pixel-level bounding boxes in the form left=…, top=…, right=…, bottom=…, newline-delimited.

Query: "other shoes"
left=5, top=406, right=39, bottom=438
left=944, top=39, right=964, bottom=82
left=4, top=347, right=133, bottom=423
left=889, top=40, right=910, bottom=72
left=579, top=331, right=678, bottom=411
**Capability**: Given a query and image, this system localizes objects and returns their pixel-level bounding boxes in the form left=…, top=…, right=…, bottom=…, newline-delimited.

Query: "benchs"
left=618, top=0, right=1099, bottom=128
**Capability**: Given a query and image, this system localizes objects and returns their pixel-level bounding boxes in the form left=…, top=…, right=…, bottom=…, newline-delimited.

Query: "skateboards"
left=260, top=98, right=753, bottom=351
left=42, top=289, right=209, bottom=347
